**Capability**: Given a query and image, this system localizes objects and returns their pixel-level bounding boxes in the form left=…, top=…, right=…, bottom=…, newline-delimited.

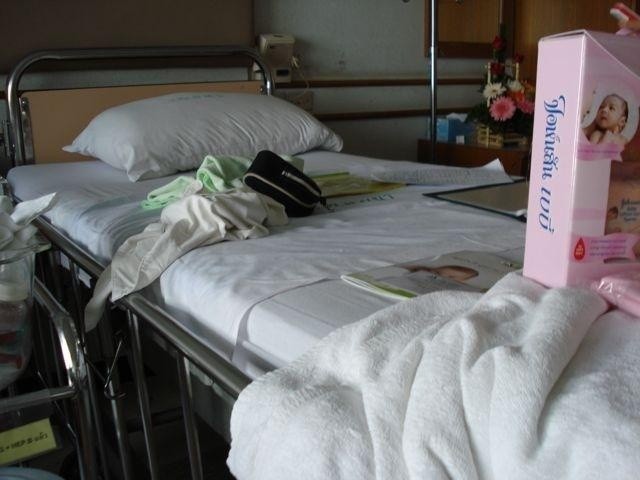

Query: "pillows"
left=59, top=90, right=346, bottom=183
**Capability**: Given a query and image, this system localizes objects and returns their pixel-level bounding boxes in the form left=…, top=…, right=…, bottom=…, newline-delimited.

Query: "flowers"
left=466, top=35, right=536, bottom=133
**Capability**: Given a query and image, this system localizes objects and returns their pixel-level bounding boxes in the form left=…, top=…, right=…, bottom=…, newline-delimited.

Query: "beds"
left=7, top=43, right=639, bottom=480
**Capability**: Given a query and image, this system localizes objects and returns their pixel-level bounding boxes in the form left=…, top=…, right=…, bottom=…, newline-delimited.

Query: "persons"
left=590, top=94, right=629, bottom=145
left=406, top=260, right=478, bottom=282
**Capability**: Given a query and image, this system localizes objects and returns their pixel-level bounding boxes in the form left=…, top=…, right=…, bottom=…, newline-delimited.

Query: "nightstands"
left=415, top=135, right=531, bottom=178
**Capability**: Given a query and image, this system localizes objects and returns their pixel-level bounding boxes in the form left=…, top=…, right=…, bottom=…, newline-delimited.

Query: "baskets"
left=475, top=61, right=528, bottom=148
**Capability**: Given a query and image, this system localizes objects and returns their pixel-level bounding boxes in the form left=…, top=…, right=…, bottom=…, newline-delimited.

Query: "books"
left=340, top=248, right=525, bottom=306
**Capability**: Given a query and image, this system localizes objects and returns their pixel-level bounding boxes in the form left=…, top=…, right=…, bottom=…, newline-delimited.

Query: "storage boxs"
left=0, top=205, right=51, bottom=388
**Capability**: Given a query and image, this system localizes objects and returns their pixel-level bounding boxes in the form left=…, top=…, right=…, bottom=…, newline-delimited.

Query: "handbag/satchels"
left=243, top=150, right=334, bottom=218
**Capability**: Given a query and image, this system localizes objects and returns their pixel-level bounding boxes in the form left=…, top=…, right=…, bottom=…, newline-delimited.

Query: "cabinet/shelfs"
left=506, top=2, right=629, bottom=76
left=424, top=0, right=511, bottom=60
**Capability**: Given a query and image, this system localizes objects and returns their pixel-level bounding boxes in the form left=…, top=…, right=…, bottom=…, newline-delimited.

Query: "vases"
left=476, top=124, right=528, bottom=144
